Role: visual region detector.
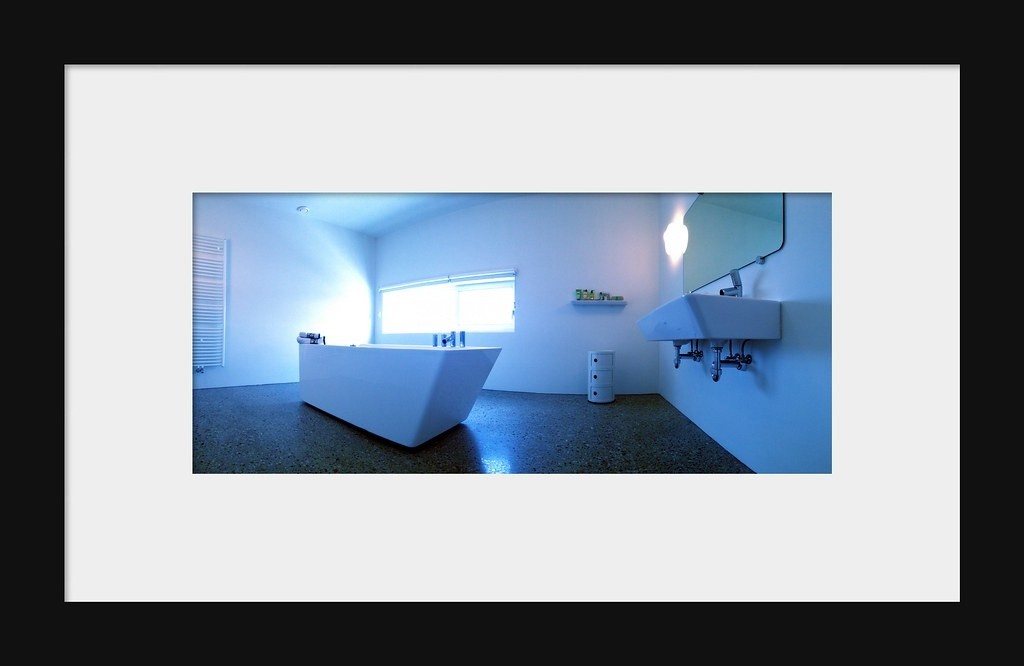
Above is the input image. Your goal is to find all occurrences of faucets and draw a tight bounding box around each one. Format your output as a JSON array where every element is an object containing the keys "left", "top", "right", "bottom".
[
  {"left": 719, "top": 268, "right": 743, "bottom": 298},
  {"left": 442, "top": 331, "right": 457, "bottom": 347}
]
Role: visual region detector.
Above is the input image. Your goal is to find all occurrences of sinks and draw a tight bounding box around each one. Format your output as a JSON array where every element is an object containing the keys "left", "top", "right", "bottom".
[{"left": 636, "top": 293, "right": 781, "bottom": 342}]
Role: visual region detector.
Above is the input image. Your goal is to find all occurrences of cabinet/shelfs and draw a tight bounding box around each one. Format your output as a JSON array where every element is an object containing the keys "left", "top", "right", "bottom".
[{"left": 587, "top": 349, "right": 616, "bottom": 403}]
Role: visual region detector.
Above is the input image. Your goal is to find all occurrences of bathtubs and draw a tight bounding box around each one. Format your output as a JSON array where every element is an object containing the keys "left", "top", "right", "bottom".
[{"left": 298, "top": 344, "right": 502, "bottom": 448}]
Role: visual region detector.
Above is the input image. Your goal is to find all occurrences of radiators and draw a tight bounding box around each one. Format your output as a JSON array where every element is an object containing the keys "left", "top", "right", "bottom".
[{"left": 192, "top": 231, "right": 227, "bottom": 374}]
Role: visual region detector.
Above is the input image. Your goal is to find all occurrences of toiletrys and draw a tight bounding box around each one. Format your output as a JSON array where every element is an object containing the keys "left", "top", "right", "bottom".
[{"left": 575, "top": 288, "right": 624, "bottom": 301}]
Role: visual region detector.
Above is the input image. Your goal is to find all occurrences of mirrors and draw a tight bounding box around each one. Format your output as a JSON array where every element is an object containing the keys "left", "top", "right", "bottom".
[{"left": 682, "top": 192, "right": 786, "bottom": 296}]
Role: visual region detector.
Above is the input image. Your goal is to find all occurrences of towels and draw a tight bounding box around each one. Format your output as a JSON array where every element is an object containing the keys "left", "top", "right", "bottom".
[{"left": 296, "top": 331, "right": 326, "bottom": 345}]
